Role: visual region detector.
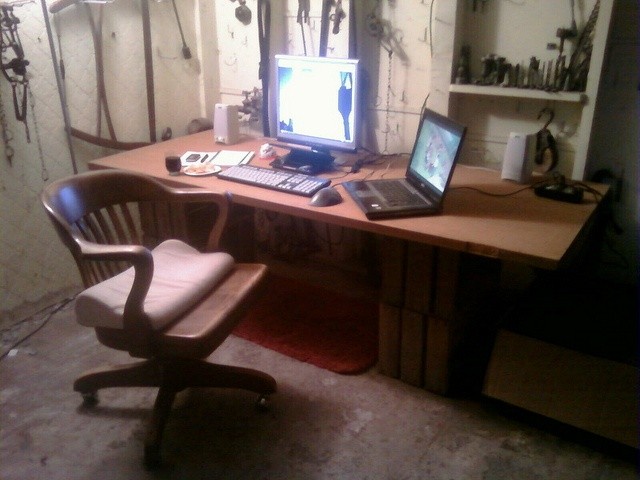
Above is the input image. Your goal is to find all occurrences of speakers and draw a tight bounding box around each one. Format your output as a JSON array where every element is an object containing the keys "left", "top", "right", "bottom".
[
  {"left": 214, "top": 103, "right": 240, "bottom": 146},
  {"left": 501, "top": 133, "right": 537, "bottom": 184}
]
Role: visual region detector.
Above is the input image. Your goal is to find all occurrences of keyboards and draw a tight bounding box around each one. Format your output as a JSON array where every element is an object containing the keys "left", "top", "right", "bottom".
[{"left": 217, "top": 164, "right": 331, "bottom": 198}]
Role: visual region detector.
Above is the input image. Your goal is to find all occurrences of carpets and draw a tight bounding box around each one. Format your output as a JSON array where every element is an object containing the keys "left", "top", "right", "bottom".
[{"left": 230, "top": 273, "right": 378, "bottom": 377}]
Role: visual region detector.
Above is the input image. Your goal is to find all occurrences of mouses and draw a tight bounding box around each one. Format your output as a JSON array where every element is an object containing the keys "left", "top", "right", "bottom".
[{"left": 310, "top": 186, "right": 342, "bottom": 207}]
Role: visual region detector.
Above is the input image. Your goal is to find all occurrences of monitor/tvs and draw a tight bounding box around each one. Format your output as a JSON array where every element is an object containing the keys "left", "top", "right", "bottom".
[{"left": 270, "top": 54, "right": 361, "bottom": 177}]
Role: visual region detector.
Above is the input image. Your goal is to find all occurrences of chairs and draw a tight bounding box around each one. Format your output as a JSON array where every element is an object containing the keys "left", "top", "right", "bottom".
[{"left": 38, "top": 169, "right": 278, "bottom": 472}]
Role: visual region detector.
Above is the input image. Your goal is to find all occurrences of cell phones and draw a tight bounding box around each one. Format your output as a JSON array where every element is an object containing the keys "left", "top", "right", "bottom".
[{"left": 186, "top": 153, "right": 200, "bottom": 162}]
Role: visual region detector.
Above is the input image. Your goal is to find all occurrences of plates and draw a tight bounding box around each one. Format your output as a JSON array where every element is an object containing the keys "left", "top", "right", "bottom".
[{"left": 182, "top": 164, "right": 221, "bottom": 176}]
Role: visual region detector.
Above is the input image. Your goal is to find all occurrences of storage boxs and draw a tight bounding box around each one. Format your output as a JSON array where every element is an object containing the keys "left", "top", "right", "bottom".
[{"left": 482, "top": 328, "right": 640, "bottom": 449}]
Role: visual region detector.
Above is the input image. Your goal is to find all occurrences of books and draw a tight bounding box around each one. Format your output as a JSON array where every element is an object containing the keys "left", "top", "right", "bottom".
[{"left": 212, "top": 150, "right": 254, "bottom": 166}]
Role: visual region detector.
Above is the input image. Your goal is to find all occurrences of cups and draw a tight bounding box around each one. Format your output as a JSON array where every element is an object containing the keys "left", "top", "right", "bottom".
[{"left": 164, "top": 152, "right": 182, "bottom": 178}]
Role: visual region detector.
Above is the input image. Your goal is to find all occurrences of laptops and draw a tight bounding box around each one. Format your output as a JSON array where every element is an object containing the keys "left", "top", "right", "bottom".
[{"left": 342, "top": 107, "right": 467, "bottom": 219}]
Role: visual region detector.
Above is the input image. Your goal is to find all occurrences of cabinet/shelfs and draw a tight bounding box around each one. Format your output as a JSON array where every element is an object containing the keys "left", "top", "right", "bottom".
[{"left": 427, "top": 0, "right": 616, "bottom": 182}]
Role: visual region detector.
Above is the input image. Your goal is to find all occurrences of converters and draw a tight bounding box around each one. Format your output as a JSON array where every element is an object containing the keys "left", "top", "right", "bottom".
[{"left": 534, "top": 183, "right": 582, "bottom": 203}]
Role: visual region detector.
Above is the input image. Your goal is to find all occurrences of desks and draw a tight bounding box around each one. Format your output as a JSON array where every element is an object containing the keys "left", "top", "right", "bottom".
[{"left": 86, "top": 126, "right": 612, "bottom": 396}]
